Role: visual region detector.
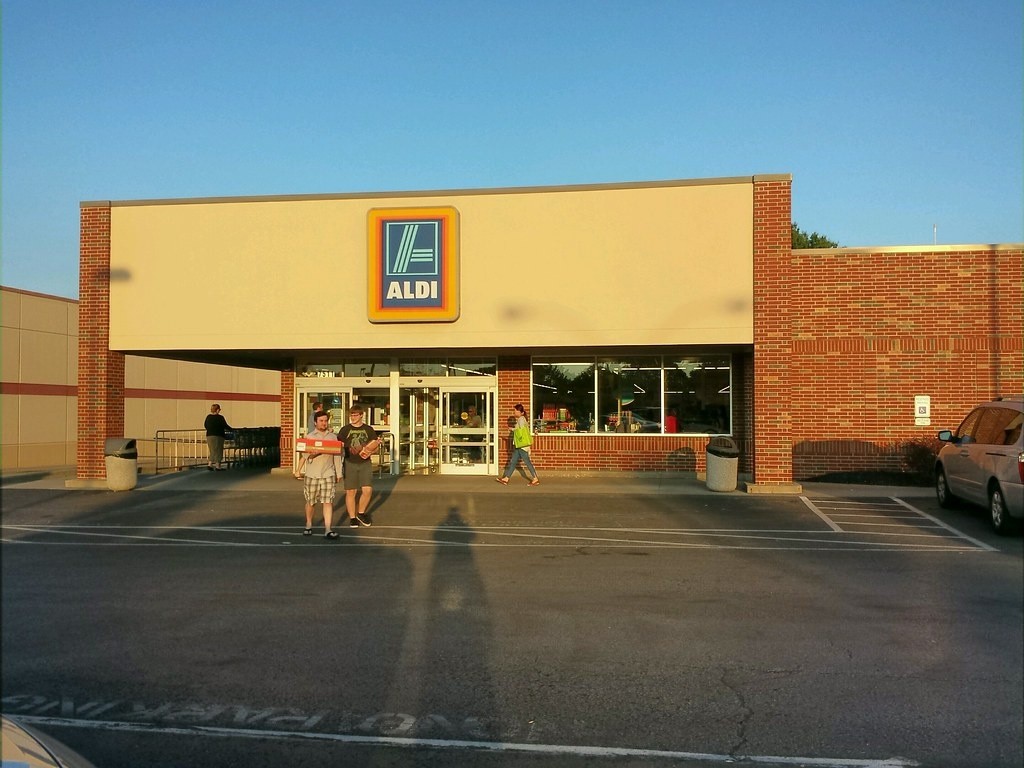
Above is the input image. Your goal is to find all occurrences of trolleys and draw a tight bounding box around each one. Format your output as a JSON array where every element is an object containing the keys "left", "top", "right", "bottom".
[{"left": 222, "top": 425, "right": 281, "bottom": 473}]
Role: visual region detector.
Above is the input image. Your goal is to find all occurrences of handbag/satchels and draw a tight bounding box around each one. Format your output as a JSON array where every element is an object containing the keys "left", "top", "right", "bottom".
[{"left": 514, "top": 426, "right": 534, "bottom": 449}]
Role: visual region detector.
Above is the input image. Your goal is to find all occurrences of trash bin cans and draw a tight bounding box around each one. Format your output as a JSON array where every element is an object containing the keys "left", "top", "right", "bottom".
[
  {"left": 706, "top": 436, "right": 739, "bottom": 492},
  {"left": 104, "top": 437, "right": 138, "bottom": 491}
]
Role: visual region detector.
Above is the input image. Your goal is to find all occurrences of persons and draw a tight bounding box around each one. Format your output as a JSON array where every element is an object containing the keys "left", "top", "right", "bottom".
[
  {"left": 338, "top": 405, "right": 376, "bottom": 529},
  {"left": 496, "top": 404, "right": 540, "bottom": 487},
  {"left": 294, "top": 402, "right": 324, "bottom": 481},
  {"left": 466, "top": 405, "right": 482, "bottom": 461},
  {"left": 301, "top": 411, "right": 344, "bottom": 538},
  {"left": 204, "top": 404, "right": 234, "bottom": 471}
]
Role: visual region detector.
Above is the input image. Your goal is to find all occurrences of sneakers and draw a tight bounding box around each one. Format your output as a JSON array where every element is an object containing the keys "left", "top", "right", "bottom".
[
  {"left": 350, "top": 518, "right": 359, "bottom": 528},
  {"left": 356, "top": 513, "right": 371, "bottom": 526}
]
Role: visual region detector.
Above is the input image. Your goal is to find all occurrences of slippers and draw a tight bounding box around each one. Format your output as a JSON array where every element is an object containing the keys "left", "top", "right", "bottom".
[
  {"left": 324, "top": 531, "right": 339, "bottom": 540},
  {"left": 293, "top": 476, "right": 304, "bottom": 481},
  {"left": 303, "top": 529, "right": 312, "bottom": 536}
]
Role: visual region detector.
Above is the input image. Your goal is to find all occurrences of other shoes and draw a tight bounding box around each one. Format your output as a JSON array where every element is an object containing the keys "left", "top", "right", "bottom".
[
  {"left": 216, "top": 468, "right": 221, "bottom": 471},
  {"left": 495, "top": 478, "right": 508, "bottom": 486},
  {"left": 527, "top": 480, "right": 540, "bottom": 486},
  {"left": 207, "top": 466, "right": 214, "bottom": 471}
]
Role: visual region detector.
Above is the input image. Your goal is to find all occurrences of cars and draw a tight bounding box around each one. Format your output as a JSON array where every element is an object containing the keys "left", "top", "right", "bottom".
[
  {"left": 933, "top": 392, "right": 1024, "bottom": 537},
  {"left": 599, "top": 409, "right": 661, "bottom": 433}
]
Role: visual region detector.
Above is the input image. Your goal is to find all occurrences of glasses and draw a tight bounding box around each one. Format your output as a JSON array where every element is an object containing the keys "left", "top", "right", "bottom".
[
  {"left": 350, "top": 414, "right": 360, "bottom": 417},
  {"left": 219, "top": 409, "right": 221, "bottom": 411}
]
